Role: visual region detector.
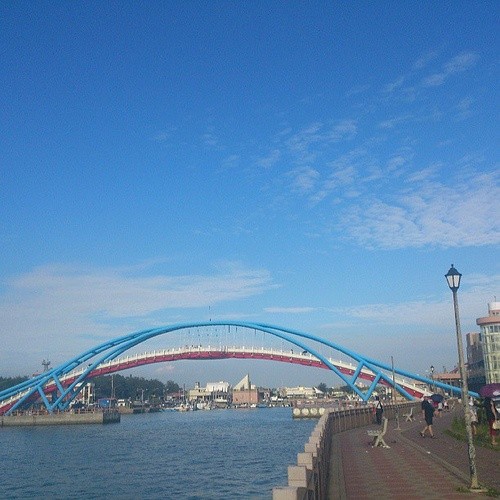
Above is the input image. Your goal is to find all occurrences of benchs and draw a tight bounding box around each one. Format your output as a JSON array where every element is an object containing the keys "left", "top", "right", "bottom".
[
  {"left": 403, "top": 406, "right": 416, "bottom": 424},
  {"left": 367, "top": 416, "right": 390, "bottom": 448}
]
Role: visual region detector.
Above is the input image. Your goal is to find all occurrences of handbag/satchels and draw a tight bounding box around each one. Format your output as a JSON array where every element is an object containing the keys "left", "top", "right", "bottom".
[
  {"left": 492, "top": 420, "right": 500, "bottom": 430},
  {"left": 373, "top": 407, "right": 376, "bottom": 414},
  {"left": 382, "top": 408, "right": 383, "bottom": 412}
]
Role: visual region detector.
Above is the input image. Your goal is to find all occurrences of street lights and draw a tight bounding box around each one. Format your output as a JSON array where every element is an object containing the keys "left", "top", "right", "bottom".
[
  {"left": 431, "top": 365, "right": 436, "bottom": 394},
  {"left": 444, "top": 263, "right": 483, "bottom": 490}
]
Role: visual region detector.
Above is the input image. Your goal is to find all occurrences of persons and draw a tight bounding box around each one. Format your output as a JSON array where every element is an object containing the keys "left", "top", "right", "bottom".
[
  {"left": 469, "top": 396, "right": 491, "bottom": 435},
  {"left": 420, "top": 393, "right": 435, "bottom": 439},
  {"left": 487, "top": 399, "right": 499, "bottom": 445},
  {"left": 432, "top": 400, "right": 449, "bottom": 418},
  {"left": 372, "top": 396, "right": 384, "bottom": 424}
]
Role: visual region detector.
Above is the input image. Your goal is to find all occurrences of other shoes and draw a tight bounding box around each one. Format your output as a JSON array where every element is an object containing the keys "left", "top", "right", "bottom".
[
  {"left": 431, "top": 435, "right": 438, "bottom": 439},
  {"left": 420, "top": 432, "right": 425, "bottom": 438}
]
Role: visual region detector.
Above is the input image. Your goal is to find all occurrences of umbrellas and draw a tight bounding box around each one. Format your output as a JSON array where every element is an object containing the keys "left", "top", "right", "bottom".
[{"left": 431, "top": 394, "right": 444, "bottom": 403}]
